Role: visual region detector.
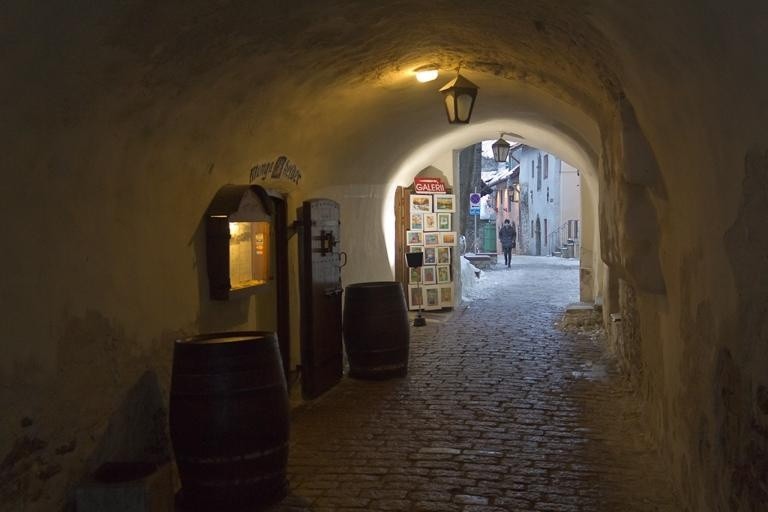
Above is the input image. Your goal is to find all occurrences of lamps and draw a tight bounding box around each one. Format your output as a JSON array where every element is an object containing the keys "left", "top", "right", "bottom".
[
  {"left": 439, "top": 65, "right": 482, "bottom": 126},
  {"left": 491, "top": 132, "right": 512, "bottom": 163}
]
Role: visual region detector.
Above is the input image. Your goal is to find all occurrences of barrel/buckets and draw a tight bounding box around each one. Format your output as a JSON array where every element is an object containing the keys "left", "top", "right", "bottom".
[
  {"left": 343, "top": 282, "right": 410, "bottom": 380},
  {"left": 169, "top": 331, "right": 290, "bottom": 512}
]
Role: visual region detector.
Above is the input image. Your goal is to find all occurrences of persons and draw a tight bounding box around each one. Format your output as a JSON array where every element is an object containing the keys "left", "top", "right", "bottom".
[{"left": 499, "top": 219, "right": 516, "bottom": 268}]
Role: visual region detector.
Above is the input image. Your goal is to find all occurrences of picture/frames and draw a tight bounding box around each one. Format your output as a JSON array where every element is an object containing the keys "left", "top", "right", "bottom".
[{"left": 405, "top": 193, "right": 459, "bottom": 313}]
